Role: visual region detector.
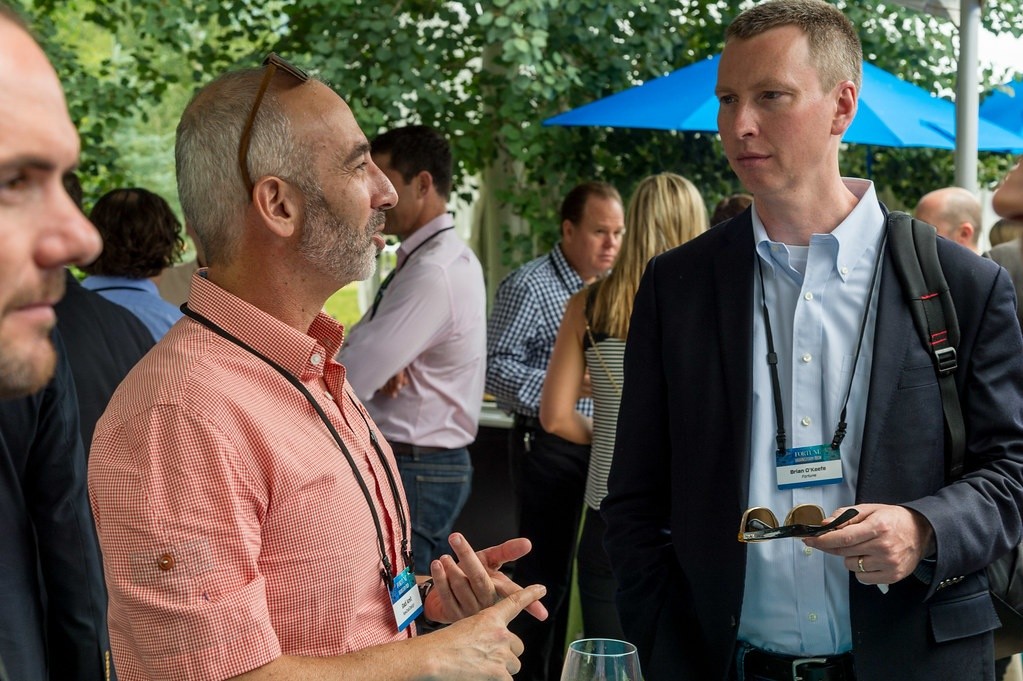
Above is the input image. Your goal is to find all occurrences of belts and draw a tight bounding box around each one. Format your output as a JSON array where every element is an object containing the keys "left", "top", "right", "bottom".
[{"left": 389, "top": 441, "right": 445, "bottom": 454}]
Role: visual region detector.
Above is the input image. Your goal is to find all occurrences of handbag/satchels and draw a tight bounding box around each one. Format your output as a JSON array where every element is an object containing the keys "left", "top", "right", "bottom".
[{"left": 886, "top": 211, "right": 1023, "bottom": 617}]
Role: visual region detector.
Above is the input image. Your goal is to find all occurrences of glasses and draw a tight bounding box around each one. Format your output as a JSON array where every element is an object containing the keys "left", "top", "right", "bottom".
[
  {"left": 737, "top": 504, "right": 860, "bottom": 543},
  {"left": 238, "top": 50, "right": 309, "bottom": 202}
]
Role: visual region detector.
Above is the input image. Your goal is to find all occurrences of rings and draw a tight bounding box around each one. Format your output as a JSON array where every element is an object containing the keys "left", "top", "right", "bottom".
[{"left": 858, "top": 556, "right": 867, "bottom": 572}]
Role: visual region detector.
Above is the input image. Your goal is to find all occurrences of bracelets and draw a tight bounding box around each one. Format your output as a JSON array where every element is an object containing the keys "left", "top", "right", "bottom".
[{"left": 420, "top": 579, "right": 434, "bottom": 602}]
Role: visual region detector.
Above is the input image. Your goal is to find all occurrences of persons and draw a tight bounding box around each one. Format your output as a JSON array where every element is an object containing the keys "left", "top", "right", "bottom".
[
  {"left": 334, "top": 127, "right": 486, "bottom": 574},
  {"left": 913, "top": 155, "right": 1023, "bottom": 329},
  {"left": 84, "top": 65, "right": 548, "bottom": 680},
  {"left": 598, "top": 0, "right": 1023, "bottom": 679},
  {"left": 0, "top": 12, "right": 187, "bottom": 680},
  {"left": 486, "top": 183, "right": 623, "bottom": 681},
  {"left": 538, "top": 172, "right": 712, "bottom": 643}
]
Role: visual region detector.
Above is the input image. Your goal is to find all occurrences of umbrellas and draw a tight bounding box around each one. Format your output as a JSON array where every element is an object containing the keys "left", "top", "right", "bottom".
[{"left": 544, "top": 54, "right": 1022, "bottom": 151}]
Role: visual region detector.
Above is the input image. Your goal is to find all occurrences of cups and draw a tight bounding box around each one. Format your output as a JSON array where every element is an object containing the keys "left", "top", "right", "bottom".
[{"left": 560, "top": 638, "right": 644, "bottom": 681}]
